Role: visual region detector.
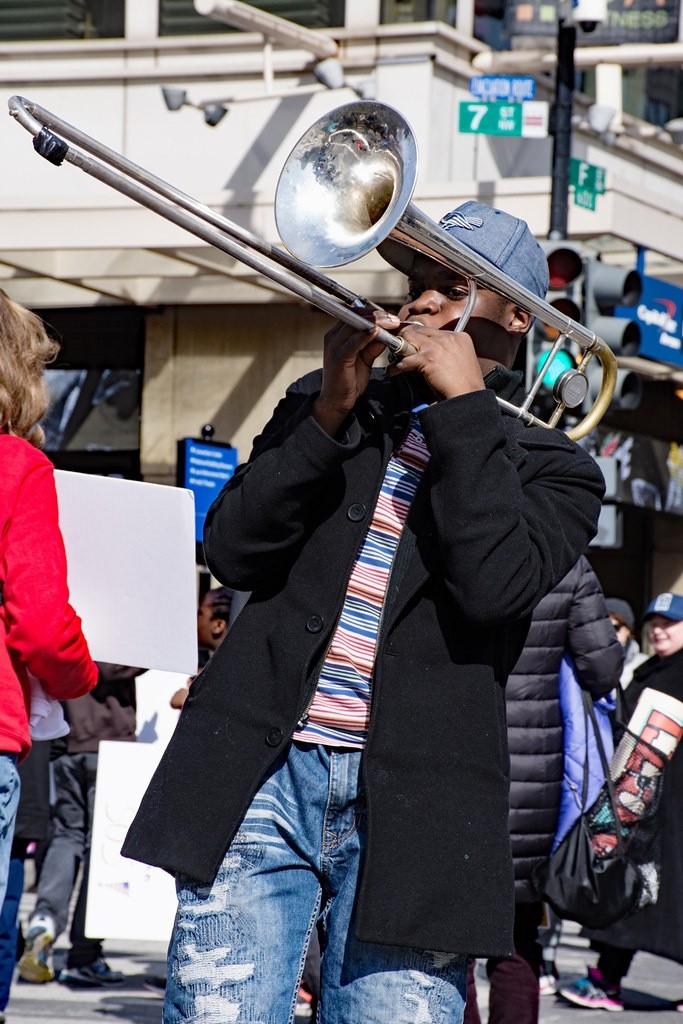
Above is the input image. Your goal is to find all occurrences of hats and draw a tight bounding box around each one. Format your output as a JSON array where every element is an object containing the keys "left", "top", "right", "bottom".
[
  {"left": 377, "top": 202, "right": 548, "bottom": 323},
  {"left": 640, "top": 591, "right": 682, "bottom": 622},
  {"left": 603, "top": 597, "right": 634, "bottom": 632}
]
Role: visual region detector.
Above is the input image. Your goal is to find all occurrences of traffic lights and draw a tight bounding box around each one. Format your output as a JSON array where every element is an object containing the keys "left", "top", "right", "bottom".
[
  {"left": 524, "top": 238, "right": 585, "bottom": 396},
  {"left": 587, "top": 449, "right": 624, "bottom": 551},
  {"left": 575, "top": 255, "right": 644, "bottom": 415}
]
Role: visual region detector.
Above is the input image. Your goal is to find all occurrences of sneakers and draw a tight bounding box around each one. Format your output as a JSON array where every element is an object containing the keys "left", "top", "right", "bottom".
[
  {"left": 58, "top": 956, "right": 128, "bottom": 986},
  {"left": 20, "top": 915, "right": 57, "bottom": 984},
  {"left": 558, "top": 965, "right": 624, "bottom": 1014}
]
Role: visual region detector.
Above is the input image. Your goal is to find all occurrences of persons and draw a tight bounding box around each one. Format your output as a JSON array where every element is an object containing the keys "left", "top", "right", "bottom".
[
  {"left": 461, "top": 555, "right": 683, "bottom": 1024},
  {"left": 120, "top": 200, "right": 607, "bottom": 1024},
  {"left": 0, "top": 281, "right": 321, "bottom": 1024}
]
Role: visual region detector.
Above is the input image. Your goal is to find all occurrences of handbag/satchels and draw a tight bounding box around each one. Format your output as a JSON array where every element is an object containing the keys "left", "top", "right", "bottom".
[{"left": 529, "top": 816, "right": 645, "bottom": 928}]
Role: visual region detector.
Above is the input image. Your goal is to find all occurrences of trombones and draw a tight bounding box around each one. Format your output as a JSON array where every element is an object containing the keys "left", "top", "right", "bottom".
[{"left": 7, "top": 95, "right": 619, "bottom": 443}]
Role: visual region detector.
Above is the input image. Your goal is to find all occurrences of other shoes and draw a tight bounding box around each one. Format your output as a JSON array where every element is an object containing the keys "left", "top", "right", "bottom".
[
  {"left": 538, "top": 974, "right": 558, "bottom": 994},
  {"left": 142, "top": 977, "right": 167, "bottom": 995}
]
